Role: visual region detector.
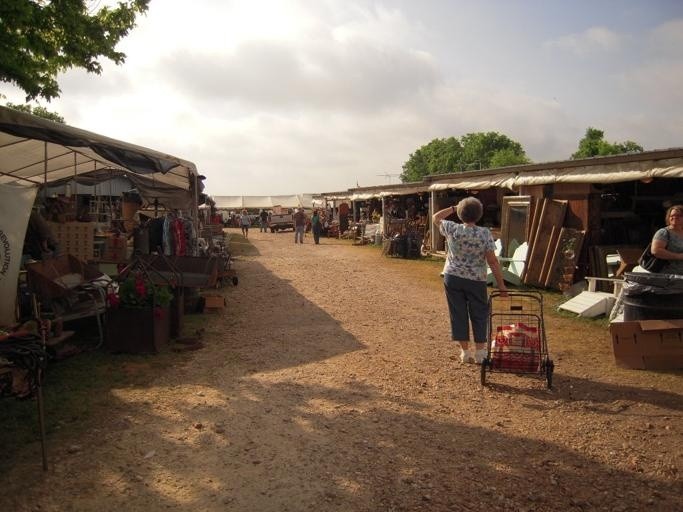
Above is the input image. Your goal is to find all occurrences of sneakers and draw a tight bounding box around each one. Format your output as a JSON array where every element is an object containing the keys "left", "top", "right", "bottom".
[
  {"left": 473, "top": 349, "right": 486, "bottom": 366},
  {"left": 459, "top": 349, "right": 470, "bottom": 363}
]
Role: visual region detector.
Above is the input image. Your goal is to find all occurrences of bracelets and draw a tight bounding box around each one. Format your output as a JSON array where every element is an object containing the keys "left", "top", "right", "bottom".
[{"left": 451, "top": 205, "right": 454, "bottom": 214}]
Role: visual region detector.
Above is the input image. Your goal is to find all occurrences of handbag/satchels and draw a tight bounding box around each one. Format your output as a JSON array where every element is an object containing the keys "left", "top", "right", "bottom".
[{"left": 638, "top": 228, "right": 671, "bottom": 273}]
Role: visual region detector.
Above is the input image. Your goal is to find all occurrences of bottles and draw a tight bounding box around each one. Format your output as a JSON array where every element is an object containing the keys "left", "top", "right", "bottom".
[{"left": 106, "top": 285, "right": 113, "bottom": 294}]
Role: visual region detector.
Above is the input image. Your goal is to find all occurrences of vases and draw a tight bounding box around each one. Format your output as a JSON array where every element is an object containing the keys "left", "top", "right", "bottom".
[{"left": 114, "top": 305, "right": 169, "bottom": 356}]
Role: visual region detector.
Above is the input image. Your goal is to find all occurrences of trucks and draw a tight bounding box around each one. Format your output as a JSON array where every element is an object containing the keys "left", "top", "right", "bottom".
[{"left": 224, "top": 207, "right": 295, "bottom": 233}]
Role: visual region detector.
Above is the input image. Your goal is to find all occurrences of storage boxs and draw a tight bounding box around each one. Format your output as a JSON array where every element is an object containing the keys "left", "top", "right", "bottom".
[
  {"left": 609, "top": 316, "right": 683, "bottom": 371},
  {"left": 199, "top": 293, "right": 224, "bottom": 314}
]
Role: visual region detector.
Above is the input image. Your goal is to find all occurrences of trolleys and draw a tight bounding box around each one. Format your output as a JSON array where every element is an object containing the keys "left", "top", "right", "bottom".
[
  {"left": 211, "top": 249, "right": 240, "bottom": 289},
  {"left": 478, "top": 288, "right": 555, "bottom": 390}
]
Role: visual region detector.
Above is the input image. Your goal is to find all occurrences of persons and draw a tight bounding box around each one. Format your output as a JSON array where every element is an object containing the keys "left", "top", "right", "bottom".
[
  {"left": 239, "top": 209, "right": 251, "bottom": 239},
  {"left": 310, "top": 210, "right": 323, "bottom": 244},
  {"left": 292, "top": 207, "right": 308, "bottom": 244},
  {"left": 25, "top": 210, "right": 67, "bottom": 260},
  {"left": 649, "top": 203, "right": 682, "bottom": 276},
  {"left": 258, "top": 209, "right": 269, "bottom": 233},
  {"left": 432, "top": 197, "right": 509, "bottom": 365}
]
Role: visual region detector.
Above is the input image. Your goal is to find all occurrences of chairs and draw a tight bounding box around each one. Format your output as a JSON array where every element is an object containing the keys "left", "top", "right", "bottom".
[{"left": 24, "top": 253, "right": 119, "bottom": 351}]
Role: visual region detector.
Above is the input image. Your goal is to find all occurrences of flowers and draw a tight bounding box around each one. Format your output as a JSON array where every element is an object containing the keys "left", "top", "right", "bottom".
[{"left": 106, "top": 267, "right": 177, "bottom": 322}]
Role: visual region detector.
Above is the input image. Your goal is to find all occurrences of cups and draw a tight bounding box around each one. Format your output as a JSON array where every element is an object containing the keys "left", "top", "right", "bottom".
[
  {"left": 52, "top": 317, "right": 62, "bottom": 337},
  {"left": 36, "top": 319, "right": 50, "bottom": 341}
]
